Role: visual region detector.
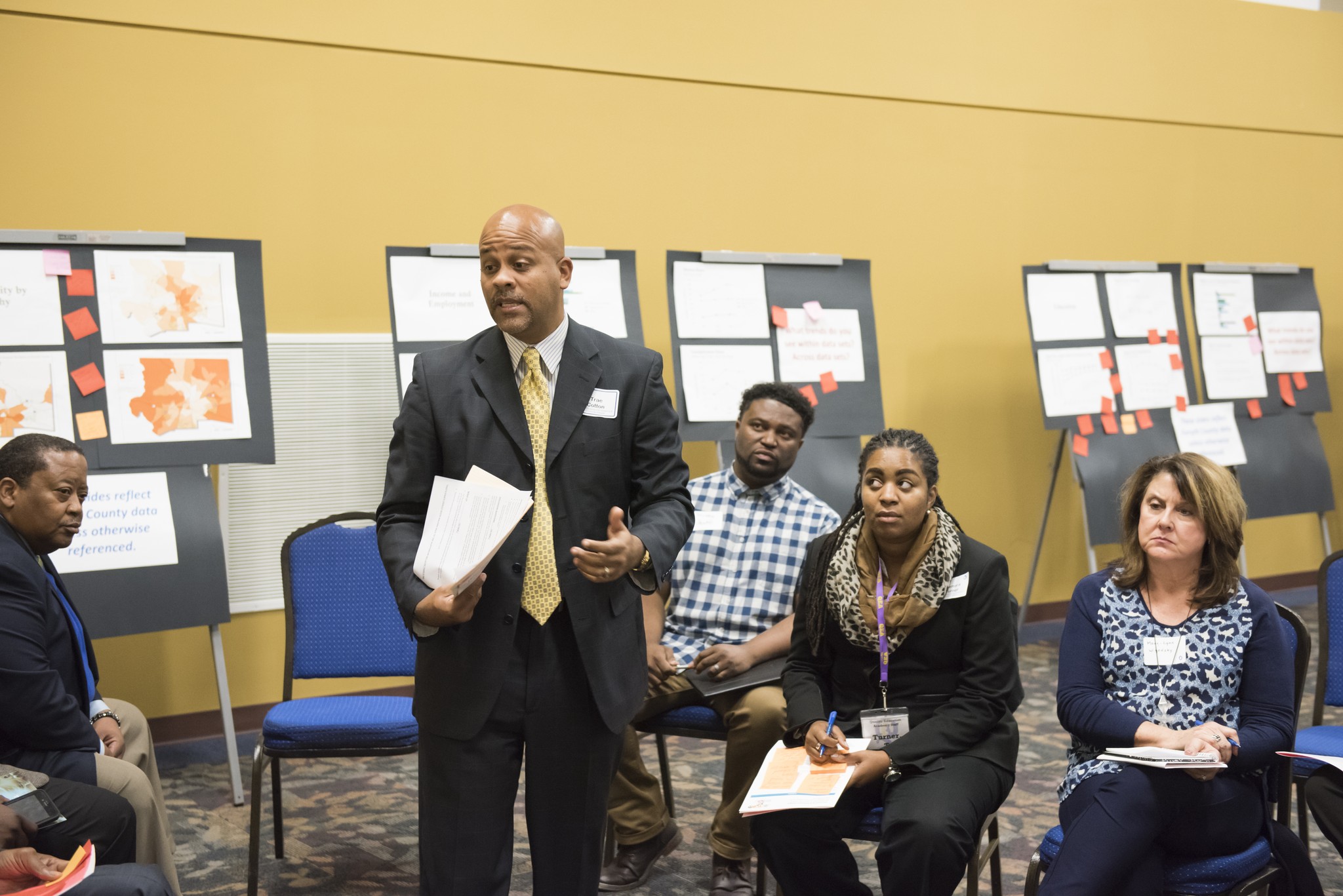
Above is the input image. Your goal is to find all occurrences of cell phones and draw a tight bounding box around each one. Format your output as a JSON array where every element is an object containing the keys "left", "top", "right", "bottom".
[{"left": 1, "top": 789, "right": 60, "bottom": 828}]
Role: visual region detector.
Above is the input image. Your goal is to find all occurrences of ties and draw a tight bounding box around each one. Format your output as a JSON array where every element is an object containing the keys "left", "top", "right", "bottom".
[
  {"left": 40, "top": 566, "right": 96, "bottom": 703},
  {"left": 515, "top": 346, "right": 563, "bottom": 625}
]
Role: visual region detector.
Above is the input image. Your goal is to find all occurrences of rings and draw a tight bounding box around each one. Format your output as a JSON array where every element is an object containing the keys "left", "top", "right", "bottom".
[
  {"left": 1212, "top": 733, "right": 1221, "bottom": 742},
  {"left": 603, "top": 565, "right": 610, "bottom": 580},
  {"left": 1200, "top": 775, "right": 1207, "bottom": 780},
  {"left": 713, "top": 663, "right": 723, "bottom": 674}
]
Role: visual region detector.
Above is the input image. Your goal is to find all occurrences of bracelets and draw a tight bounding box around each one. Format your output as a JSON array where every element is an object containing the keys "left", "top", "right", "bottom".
[{"left": 89, "top": 710, "right": 121, "bottom": 729}]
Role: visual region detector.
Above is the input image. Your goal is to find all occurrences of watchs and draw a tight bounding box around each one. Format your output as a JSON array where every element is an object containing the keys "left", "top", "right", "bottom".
[
  {"left": 632, "top": 550, "right": 652, "bottom": 573},
  {"left": 883, "top": 755, "right": 904, "bottom": 782}
]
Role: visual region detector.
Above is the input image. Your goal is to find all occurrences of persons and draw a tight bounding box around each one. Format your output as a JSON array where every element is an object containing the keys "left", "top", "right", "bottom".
[
  {"left": 746, "top": 430, "right": 1024, "bottom": 895},
  {"left": 0, "top": 433, "right": 183, "bottom": 893},
  {"left": 606, "top": 380, "right": 845, "bottom": 895},
  {"left": 0, "top": 761, "right": 138, "bottom": 863},
  {"left": 1032, "top": 448, "right": 1301, "bottom": 896},
  {"left": 371, "top": 200, "right": 698, "bottom": 894},
  {"left": 0, "top": 844, "right": 179, "bottom": 896}
]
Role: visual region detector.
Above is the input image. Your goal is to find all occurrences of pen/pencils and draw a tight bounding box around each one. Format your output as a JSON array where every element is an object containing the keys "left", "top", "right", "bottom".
[
  {"left": 819, "top": 711, "right": 837, "bottom": 758},
  {"left": 1194, "top": 719, "right": 1241, "bottom": 747},
  {"left": 670, "top": 664, "right": 696, "bottom": 668}
]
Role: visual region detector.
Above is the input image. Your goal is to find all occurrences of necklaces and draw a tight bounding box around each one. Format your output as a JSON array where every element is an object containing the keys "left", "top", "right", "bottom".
[{"left": 1146, "top": 582, "right": 1197, "bottom": 714}]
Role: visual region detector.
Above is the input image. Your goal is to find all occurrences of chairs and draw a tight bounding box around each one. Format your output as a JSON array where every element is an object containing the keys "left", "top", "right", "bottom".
[
  {"left": 754, "top": 596, "right": 1024, "bottom": 895},
  {"left": 245, "top": 514, "right": 421, "bottom": 896},
  {"left": 1001, "top": 602, "right": 1312, "bottom": 895},
  {"left": 645, "top": 699, "right": 734, "bottom": 814},
  {"left": 1279, "top": 547, "right": 1343, "bottom": 850}
]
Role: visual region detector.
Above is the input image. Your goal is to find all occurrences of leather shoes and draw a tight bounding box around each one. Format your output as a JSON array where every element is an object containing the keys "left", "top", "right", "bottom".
[
  {"left": 709, "top": 850, "right": 754, "bottom": 896},
  {"left": 597, "top": 817, "right": 683, "bottom": 890}
]
